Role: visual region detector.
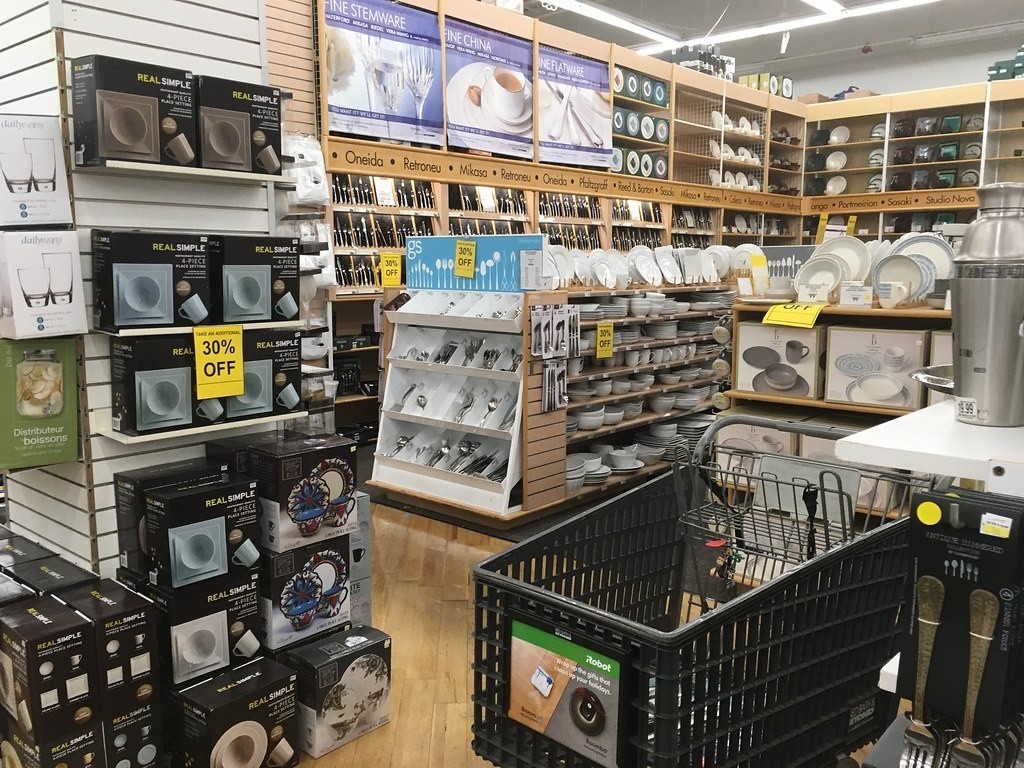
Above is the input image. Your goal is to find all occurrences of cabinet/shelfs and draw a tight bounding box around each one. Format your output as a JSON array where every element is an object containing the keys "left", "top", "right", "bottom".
[
  {"left": 294, "top": 0, "right": 1024, "bottom": 453},
  {"left": 709, "top": 279, "right": 955, "bottom": 532},
  {"left": 362, "top": 276, "right": 736, "bottom": 536},
  {"left": 63, "top": 54, "right": 311, "bottom": 447}
]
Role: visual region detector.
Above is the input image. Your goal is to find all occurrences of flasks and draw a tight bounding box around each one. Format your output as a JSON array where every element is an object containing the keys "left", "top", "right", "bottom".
[{"left": 948, "top": 177, "right": 1024, "bottom": 430}]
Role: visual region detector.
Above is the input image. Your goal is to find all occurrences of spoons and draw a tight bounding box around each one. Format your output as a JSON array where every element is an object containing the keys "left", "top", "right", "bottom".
[{"left": 332, "top": 173, "right": 530, "bottom": 487}]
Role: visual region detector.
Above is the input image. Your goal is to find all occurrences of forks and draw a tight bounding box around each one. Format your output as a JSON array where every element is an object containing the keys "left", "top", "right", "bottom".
[{"left": 894, "top": 572, "right": 1024, "bottom": 768}]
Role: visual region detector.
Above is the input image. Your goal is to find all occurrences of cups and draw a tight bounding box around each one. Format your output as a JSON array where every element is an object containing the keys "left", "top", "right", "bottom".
[
  {"left": 482, "top": 63, "right": 527, "bottom": 120},
  {"left": 6, "top": 72, "right": 392, "bottom": 768}
]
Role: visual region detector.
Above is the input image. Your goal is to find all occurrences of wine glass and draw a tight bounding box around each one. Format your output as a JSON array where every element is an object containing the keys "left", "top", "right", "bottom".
[{"left": 357, "top": 32, "right": 436, "bottom": 141}]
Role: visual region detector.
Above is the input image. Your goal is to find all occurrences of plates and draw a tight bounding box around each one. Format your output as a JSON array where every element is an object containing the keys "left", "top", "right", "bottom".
[
  {"left": 537, "top": 66, "right": 984, "bottom": 496},
  {"left": 447, "top": 60, "right": 534, "bottom": 159}
]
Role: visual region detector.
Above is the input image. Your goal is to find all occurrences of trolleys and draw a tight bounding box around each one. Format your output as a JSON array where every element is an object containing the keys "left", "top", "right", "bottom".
[{"left": 467, "top": 412, "right": 933, "bottom": 768}]
